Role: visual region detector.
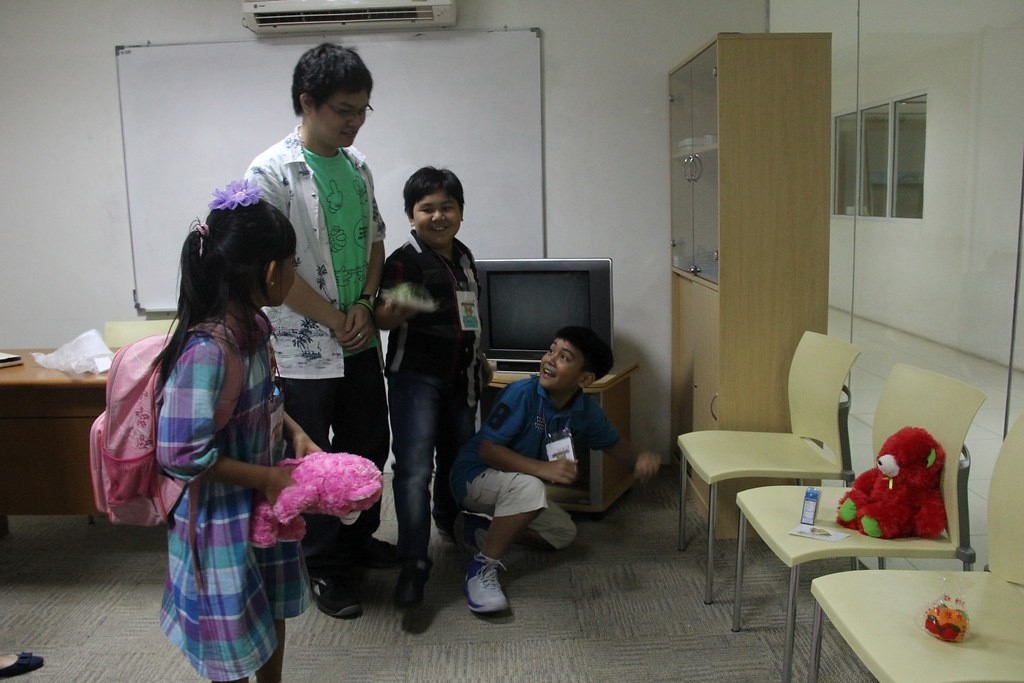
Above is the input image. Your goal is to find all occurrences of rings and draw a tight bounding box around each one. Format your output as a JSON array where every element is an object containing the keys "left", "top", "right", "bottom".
[{"left": 358, "top": 333, "right": 364, "bottom": 340}]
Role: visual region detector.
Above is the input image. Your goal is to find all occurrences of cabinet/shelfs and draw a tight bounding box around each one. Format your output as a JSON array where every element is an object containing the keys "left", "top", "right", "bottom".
[
  {"left": 669, "top": 32, "right": 831, "bottom": 540},
  {"left": 0, "top": 347, "right": 120, "bottom": 540}
]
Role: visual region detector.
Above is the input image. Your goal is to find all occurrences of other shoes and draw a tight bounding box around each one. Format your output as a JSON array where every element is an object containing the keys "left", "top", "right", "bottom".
[
  {"left": 0, "top": 651, "right": 43, "bottom": 677},
  {"left": 435, "top": 518, "right": 455, "bottom": 538},
  {"left": 395, "top": 560, "right": 428, "bottom": 609}
]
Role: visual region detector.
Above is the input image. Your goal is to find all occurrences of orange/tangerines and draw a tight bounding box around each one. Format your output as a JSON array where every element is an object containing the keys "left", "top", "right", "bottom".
[{"left": 926, "top": 606, "right": 967, "bottom": 641}]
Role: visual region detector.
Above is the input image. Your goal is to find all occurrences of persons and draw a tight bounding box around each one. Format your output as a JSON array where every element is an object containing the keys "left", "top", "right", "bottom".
[
  {"left": 454, "top": 327, "right": 659, "bottom": 613},
  {"left": 378, "top": 168, "right": 490, "bottom": 609},
  {"left": 160, "top": 180, "right": 323, "bottom": 683},
  {"left": 245, "top": 44, "right": 408, "bottom": 617},
  {"left": 0, "top": 651, "right": 44, "bottom": 678}
]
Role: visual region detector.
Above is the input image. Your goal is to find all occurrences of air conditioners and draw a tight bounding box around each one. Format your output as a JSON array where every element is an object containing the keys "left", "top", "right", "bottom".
[{"left": 242, "top": 0, "right": 456, "bottom": 34}]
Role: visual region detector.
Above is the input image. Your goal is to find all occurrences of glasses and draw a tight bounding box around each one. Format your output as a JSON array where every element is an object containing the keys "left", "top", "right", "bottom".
[{"left": 325, "top": 101, "right": 374, "bottom": 117}]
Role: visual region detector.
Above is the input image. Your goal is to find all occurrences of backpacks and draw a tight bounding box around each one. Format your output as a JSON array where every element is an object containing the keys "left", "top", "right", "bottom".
[{"left": 89, "top": 315, "right": 243, "bottom": 528}]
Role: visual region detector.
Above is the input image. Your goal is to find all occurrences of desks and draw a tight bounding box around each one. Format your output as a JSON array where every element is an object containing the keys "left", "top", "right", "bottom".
[{"left": 479, "top": 358, "right": 641, "bottom": 511}]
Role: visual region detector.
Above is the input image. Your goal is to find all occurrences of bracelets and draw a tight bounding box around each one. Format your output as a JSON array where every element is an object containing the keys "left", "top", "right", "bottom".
[{"left": 356, "top": 301, "right": 374, "bottom": 318}]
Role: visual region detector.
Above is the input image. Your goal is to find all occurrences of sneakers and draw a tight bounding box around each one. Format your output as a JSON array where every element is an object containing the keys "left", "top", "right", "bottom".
[
  {"left": 351, "top": 539, "right": 401, "bottom": 568},
  {"left": 465, "top": 556, "right": 508, "bottom": 612},
  {"left": 310, "top": 573, "right": 361, "bottom": 617},
  {"left": 454, "top": 511, "right": 480, "bottom": 558}
]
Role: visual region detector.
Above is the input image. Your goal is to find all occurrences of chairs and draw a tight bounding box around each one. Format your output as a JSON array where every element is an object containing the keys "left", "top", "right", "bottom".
[
  {"left": 88, "top": 320, "right": 180, "bottom": 523},
  {"left": 678, "top": 331, "right": 861, "bottom": 605},
  {"left": 731, "top": 364, "right": 988, "bottom": 683},
  {"left": 806, "top": 411, "right": 1024, "bottom": 683}
]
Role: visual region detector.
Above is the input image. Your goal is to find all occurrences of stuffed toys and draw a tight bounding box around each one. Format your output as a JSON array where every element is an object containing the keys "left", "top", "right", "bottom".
[
  {"left": 839, "top": 426, "right": 948, "bottom": 538},
  {"left": 249, "top": 452, "right": 385, "bottom": 548}
]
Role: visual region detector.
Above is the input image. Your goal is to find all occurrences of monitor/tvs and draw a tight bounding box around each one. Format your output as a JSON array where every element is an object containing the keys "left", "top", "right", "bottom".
[{"left": 474, "top": 257, "right": 614, "bottom": 374}]
